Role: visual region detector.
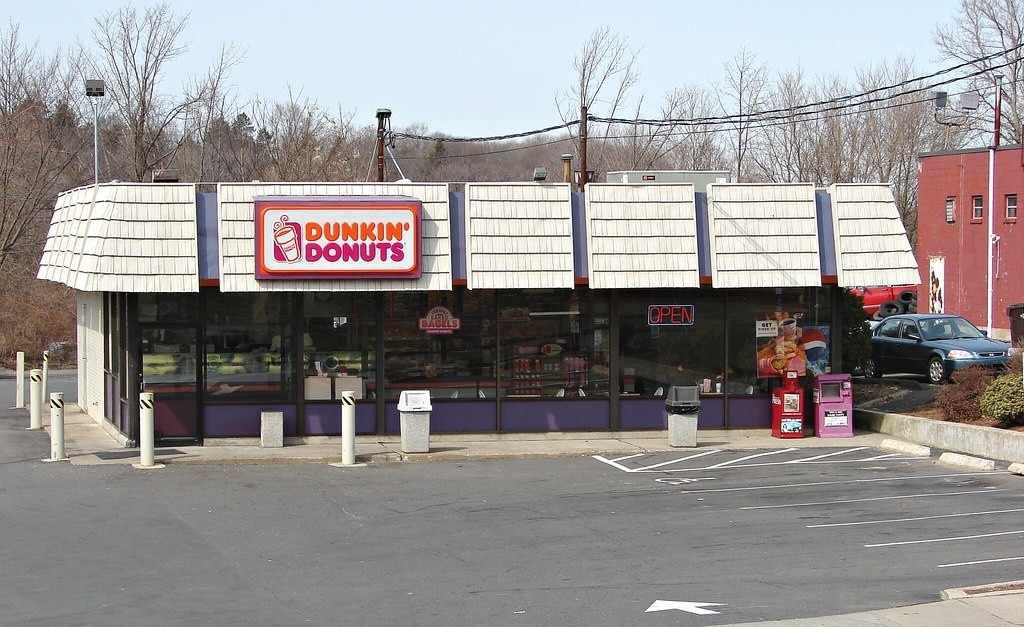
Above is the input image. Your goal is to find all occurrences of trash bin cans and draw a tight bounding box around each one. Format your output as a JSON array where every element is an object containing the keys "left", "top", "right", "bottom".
[
  {"left": 397, "top": 388, "right": 432, "bottom": 456},
  {"left": 1006, "top": 304, "right": 1024, "bottom": 348},
  {"left": 666, "top": 385, "right": 703, "bottom": 452}
]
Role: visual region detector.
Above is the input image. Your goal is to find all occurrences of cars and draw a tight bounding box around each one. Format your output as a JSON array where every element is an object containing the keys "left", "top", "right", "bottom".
[
  {"left": 863, "top": 314, "right": 1016, "bottom": 384},
  {"left": 848, "top": 284, "right": 917, "bottom": 315}
]
